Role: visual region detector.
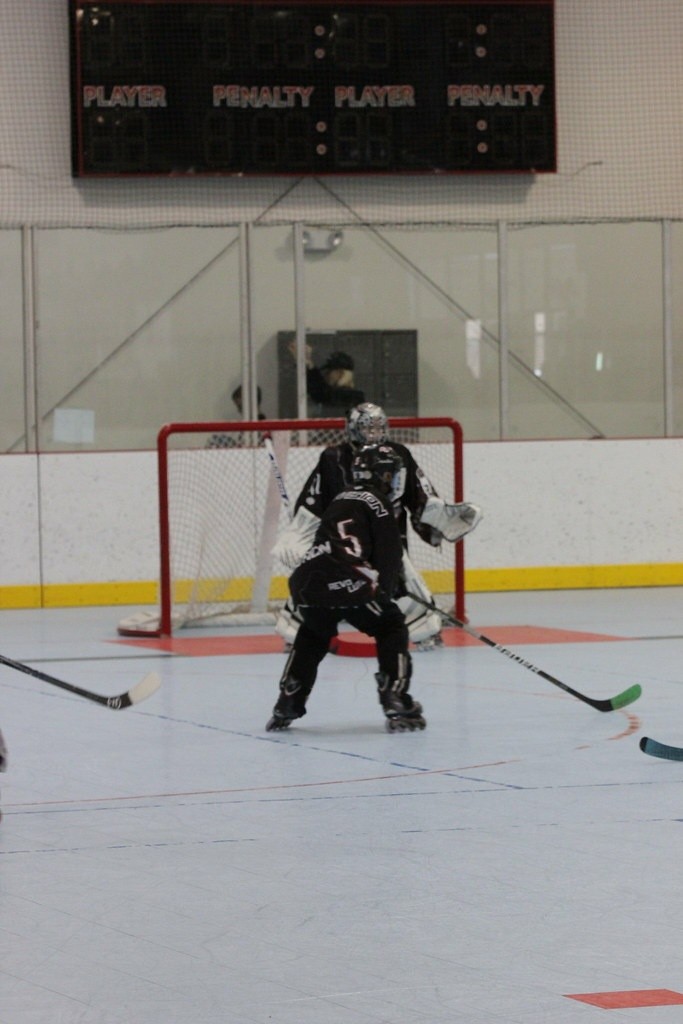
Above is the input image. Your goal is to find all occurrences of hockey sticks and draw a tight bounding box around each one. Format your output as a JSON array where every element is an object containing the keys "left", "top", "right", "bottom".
[
  {"left": 404, "top": 590, "right": 643, "bottom": 714},
  {"left": 1, "top": 655, "right": 163, "bottom": 712},
  {"left": 638, "top": 735, "right": 683, "bottom": 762},
  {"left": 260, "top": 430, "right": 297, "bottom": 526}
]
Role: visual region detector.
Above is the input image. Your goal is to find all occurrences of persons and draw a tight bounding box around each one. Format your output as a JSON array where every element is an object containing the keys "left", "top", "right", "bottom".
[
  {"left": 207, "top": 384, "right": 272, "bottom": 450},
  {"left": 264, "top": 444, "right": 426, "bottom": 733},
  {"left": 273, "top": 401, "right": 481, "bottom": 650},
  {"left": 289, "top": 339, "right": 364, "bottom": 443}
]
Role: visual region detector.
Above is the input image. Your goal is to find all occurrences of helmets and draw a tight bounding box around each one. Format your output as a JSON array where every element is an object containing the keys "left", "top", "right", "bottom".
[
  {"left": 350, "top": 444, "right": 406, "bottom": 503},
  {"left": 343, "top": 402, "right": 390, "bottom": 451}
]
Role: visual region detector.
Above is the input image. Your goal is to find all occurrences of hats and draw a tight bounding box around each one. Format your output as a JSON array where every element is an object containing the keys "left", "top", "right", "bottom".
[{"left": 317, "top": 350, "right": 354, "bottom": 373}]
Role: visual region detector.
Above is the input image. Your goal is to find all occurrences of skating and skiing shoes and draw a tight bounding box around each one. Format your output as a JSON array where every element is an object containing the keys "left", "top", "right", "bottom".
[
  {"left": 374, "top": 672, "right": 426, "bottom": 732},
  {"left": 266, "top": 679, "right": 312, "bottom": 732}
]
